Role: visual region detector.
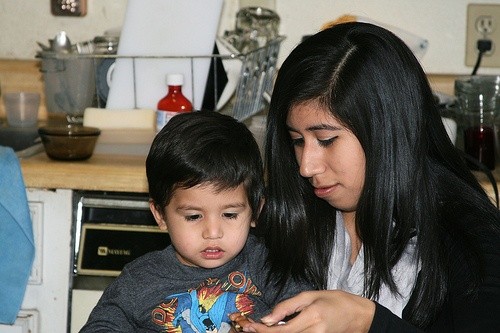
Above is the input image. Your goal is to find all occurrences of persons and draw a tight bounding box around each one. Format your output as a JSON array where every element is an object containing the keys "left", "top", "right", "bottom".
[
  {"left": 78, "top": 111, "right": 318, "bottom": 333},
  {"left": 242, "top": 21, "right": 499, "bottom": 333}
]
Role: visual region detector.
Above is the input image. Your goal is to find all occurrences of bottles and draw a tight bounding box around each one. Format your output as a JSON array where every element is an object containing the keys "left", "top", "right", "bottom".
[{"left": 153, "top": 74, "right": 193, "bottom": 136}]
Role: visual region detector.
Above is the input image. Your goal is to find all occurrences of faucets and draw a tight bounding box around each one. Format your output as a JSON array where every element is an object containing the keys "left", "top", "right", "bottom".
[{"left": 433, "top": 92, "right": 500, "bottom": 168}]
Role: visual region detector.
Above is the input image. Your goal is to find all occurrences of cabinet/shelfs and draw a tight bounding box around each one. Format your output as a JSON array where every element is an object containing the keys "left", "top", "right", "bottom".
[{"left": 0, "top": 164, "right": 172, "bottom": 333}]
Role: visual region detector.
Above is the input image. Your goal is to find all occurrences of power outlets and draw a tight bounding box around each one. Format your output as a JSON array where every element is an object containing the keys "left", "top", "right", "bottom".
[{"left": 464, "top": 3, "right": 500, "bottom": 67}]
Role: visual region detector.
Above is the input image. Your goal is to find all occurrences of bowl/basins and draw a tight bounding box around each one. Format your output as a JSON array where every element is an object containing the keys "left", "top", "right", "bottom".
[
  {"left": 39, "top": 126, "right": 102, "bottom": 162},
  {"left": 2, "top": 93, "right": 41, "bottom": 127}
]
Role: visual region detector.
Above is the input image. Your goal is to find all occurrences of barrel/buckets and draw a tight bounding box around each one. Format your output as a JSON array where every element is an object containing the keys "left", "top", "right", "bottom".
[{"left": 36, "top": 52, "right": 103, "bottom": 117}]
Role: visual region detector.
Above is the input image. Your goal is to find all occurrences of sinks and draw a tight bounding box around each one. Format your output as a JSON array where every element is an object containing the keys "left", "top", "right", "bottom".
[{"left": 1, "top": 129, "right": 43, "bottom": 152}]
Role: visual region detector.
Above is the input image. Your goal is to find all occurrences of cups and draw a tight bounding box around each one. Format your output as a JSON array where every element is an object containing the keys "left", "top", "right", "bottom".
[{"left": 450, "top": 76, "right": 500, "bottom": 172}]
